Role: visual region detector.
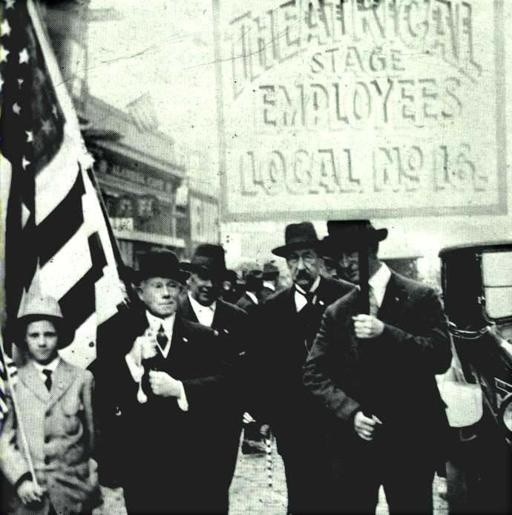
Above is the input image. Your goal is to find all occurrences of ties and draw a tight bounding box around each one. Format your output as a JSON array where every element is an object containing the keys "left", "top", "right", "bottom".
[
  {"left": 157, "top": 325, "right": 167, "bottom": 351},
  {"left": 294, "top": 288, "right": 316, "bottom": 320},
  {"left": 43, "top": 369, "right": 51, "bottom": 391},
  {"left": 367, "top": 287, "right": 379, "bottom": 316}
]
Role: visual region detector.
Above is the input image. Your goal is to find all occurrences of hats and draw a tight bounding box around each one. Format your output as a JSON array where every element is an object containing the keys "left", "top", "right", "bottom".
[
  {"left": 7, "top": 295, "right": 75, "bottom": 350},
  {"left": 130, "top": 251, "right": 191, "bottom": 282},
  {"left": 246, "top": 270, "right": 264, "bottom": 285},
  {"left": 180, "top": 244, "right": 237, "bottom": 279},
  {"left": 272, "top": 222, "right": 322, "bottom": 257},
  {"left": 312, "top": 220, "right": 388, "bottom": 256},
  {"left": 261, "top": 263, "right": 281, "bottom": 273}
]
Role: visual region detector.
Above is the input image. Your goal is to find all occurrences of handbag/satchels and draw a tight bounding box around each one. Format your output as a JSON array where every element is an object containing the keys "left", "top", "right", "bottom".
[{"left": 436, "top": 366, "right": 484, "bottom": 428}]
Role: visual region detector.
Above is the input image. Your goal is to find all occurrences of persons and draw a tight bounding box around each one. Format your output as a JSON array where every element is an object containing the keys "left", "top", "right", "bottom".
[{"left": 1, "top": 218, "right": 511, "bottom": 515}]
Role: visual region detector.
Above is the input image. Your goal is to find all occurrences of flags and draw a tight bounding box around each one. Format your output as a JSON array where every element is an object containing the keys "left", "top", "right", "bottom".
[{"left": 0, "top": 0, "right": 126, "bottom": 381}]
